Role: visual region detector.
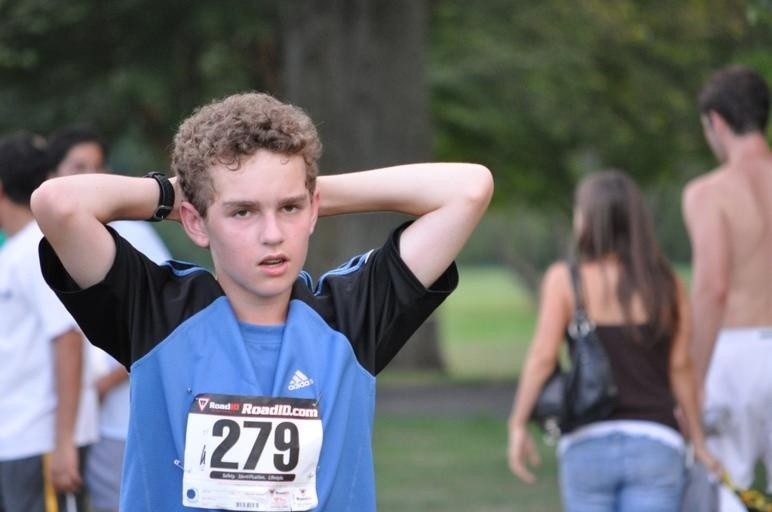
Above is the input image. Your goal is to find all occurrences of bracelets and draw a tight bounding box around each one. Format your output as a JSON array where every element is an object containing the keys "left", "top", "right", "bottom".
[{"left": 140, "top": 170, "right": 175, "bottom": 223}]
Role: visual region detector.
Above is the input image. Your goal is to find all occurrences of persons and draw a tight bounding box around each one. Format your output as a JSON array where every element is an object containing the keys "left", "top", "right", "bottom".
[
  {"left": 672, "top": 65, "right": 770, "bottom": 510},
  {"left": 1, "top": 125, "right": 102, "bottom": 511},
  {"left": 29, "top": 89, "right": 495, "bottom": 512},
  {"left": 40, "top": 125, "right": 174, "bottom": 512},
  {"left": 503, "top": 167, "right": 727, "bottom": 511}
]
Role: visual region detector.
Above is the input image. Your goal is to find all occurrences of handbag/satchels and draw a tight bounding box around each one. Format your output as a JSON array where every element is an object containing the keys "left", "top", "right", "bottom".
[{"left": 526, "top": 257, "right": 611, "bottom": 430}]
